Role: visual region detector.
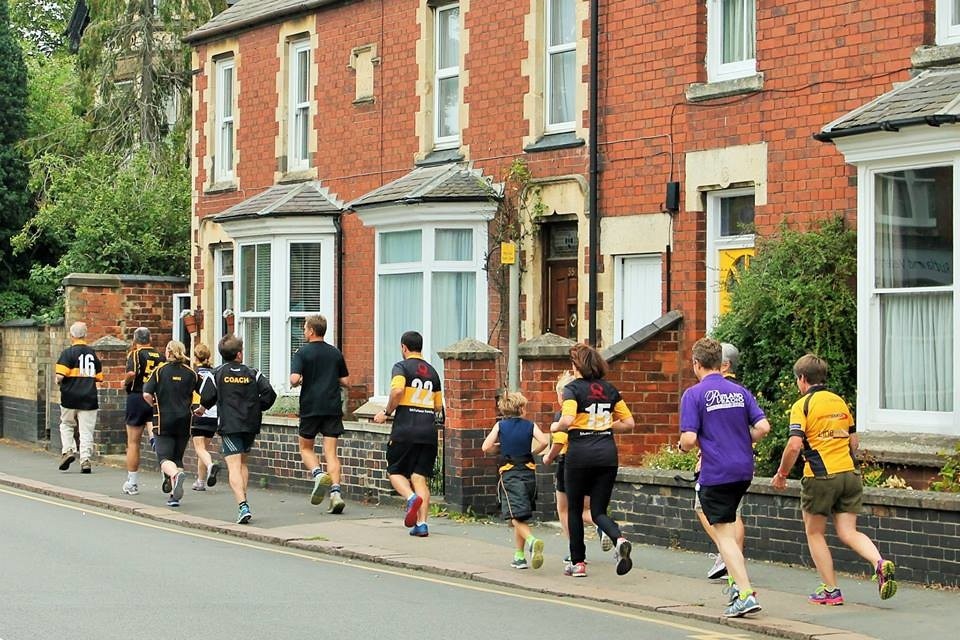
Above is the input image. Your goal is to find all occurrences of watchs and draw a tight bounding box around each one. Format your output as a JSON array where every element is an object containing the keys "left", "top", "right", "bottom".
[
  {"left": 677, "top": 442, "right": 688, "bottom": 454},
  {"left": 382, "top": 407, "right": 392, "bottom": 418}
]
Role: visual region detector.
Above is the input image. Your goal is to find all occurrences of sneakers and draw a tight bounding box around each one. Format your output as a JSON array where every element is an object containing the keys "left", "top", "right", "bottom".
[
  {"left": 595, "top": 526, "right": 612, "bottom": 552},
  {"left": 871, "top": 559, "right": 898, "bottom": 600},
  {"left": 528, "top": 538, "right": 545, "bottom": 570},
  {"left": 123, "top": 481, "right": 139, "bottom": 495},
  {"left": 162, "top": 472, "right": 172, "bottom": 494},
  {"left": 409, "top": 523, "right": 429, "bottom": 537},
  {"left": 808, "top": 582, "right": 844, "bottom": 606},
  {"left": 172, "top": 472, "right": 185, "bottom": 500},
  {"left": 59, "top": 451, "right": 75, "bottom": 470},
  {"left": 562, "top": 555, "right": 587, "bottom": 564},
  {"left": 326, "top": 494, "right": 345, "bottom": 514},
  {"left": 81, "top": 461, "right": 91, "bottom": 473},
  {"left": 613, "top": 538, "right": 633, "bottom": 576},
  {"left": 206, "top": 464, "right": 219, "bottom": 487},
  {"left": 192, "top": 480, "right": 205, "bottom": 491},
  {"left": 310, "top": 474, "right": 332, "bottom": 505},
  {"left": 510, "top": 556, "right": 527, "bottom": 569},
  {"left": 236, "top": 504, "right": 252, "bottom": 524},
  {"left": 564, "top": 561, "right": 587, "bottom": 577},
  {"left": 722, "top": 589, "right": 762, "bottom": 618},
  {"left": 723, "top": 576, "right": 739, "bottom": 607},
  {"left": 166, "top": 494, "right": 180, "bottom": 506},
  {"left": 707, "top": 553, "right": 728, "bottom": 580},
  {"left": 404, "top": 494, "right": 423, "bottom": 527}
]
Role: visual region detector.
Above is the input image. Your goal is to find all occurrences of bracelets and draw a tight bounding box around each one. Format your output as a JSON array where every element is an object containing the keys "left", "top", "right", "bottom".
[{"left": 776, "top": 466, "right": 789, "bottom": 479}]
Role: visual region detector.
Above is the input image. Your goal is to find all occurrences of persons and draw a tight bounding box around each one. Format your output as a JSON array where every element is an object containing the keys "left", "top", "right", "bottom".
[
  {"left": 190, "top": 342, "right": 222, "bottom": 491},
  {"left": 479, "top": 390, "right": 550, "bottom": 571},
  {"left": 121, "top": 323, "right": 174, "bottom": 495},
  {"left": 54, "top": 321, "right": 102, "bottom": 474},
  {"left": 142, "top": 339, "right": 206, "bottom": 505},
  {"left": 540, "top": 371, "right": 616, "bottom": 564},
  {"left": 372, "top": 329, "right": 446, "bottom": 537},
  {"left": 290, "top": 313, "right": 352, "bottom": 514},
  {"left": 673, "top": 336, "right": 775, "bottom": 618},
  {"left": 548, "top": 342, "right": 635, "bottom": 578},
  {"left": 767, "top": 353, "right": 900, "bottom": 608},
  {"left": 691, "top": 341, "right": 748, "bottom": 586},
  {"left": 197, "top": 334, "right": 278, "bottom": 525}
]
